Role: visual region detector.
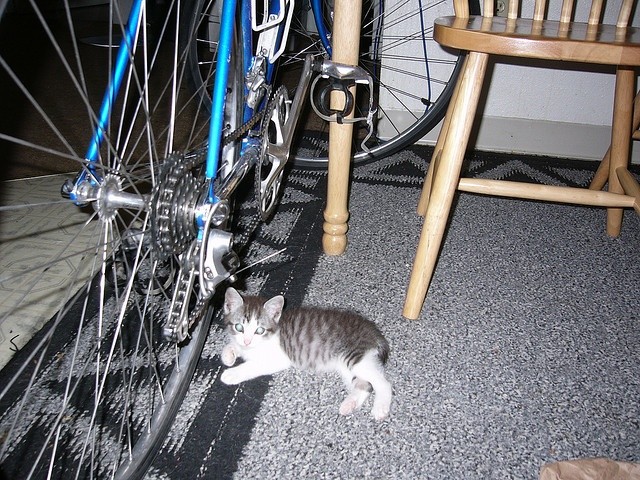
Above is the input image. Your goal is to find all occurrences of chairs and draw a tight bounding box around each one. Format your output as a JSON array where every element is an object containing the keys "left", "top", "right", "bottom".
[{"left": 400, "top": 0, "right": 639, "bottom": 320}]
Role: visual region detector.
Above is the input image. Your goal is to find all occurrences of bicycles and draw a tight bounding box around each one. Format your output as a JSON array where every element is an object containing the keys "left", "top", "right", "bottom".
[{"left": 0, "top": 0, "right": 487, "bottom": 480}]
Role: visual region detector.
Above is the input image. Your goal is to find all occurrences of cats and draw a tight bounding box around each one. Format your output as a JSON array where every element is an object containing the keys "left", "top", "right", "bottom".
[{"left": 220, "top": 286, "right": 393, "bottom": 424}]
